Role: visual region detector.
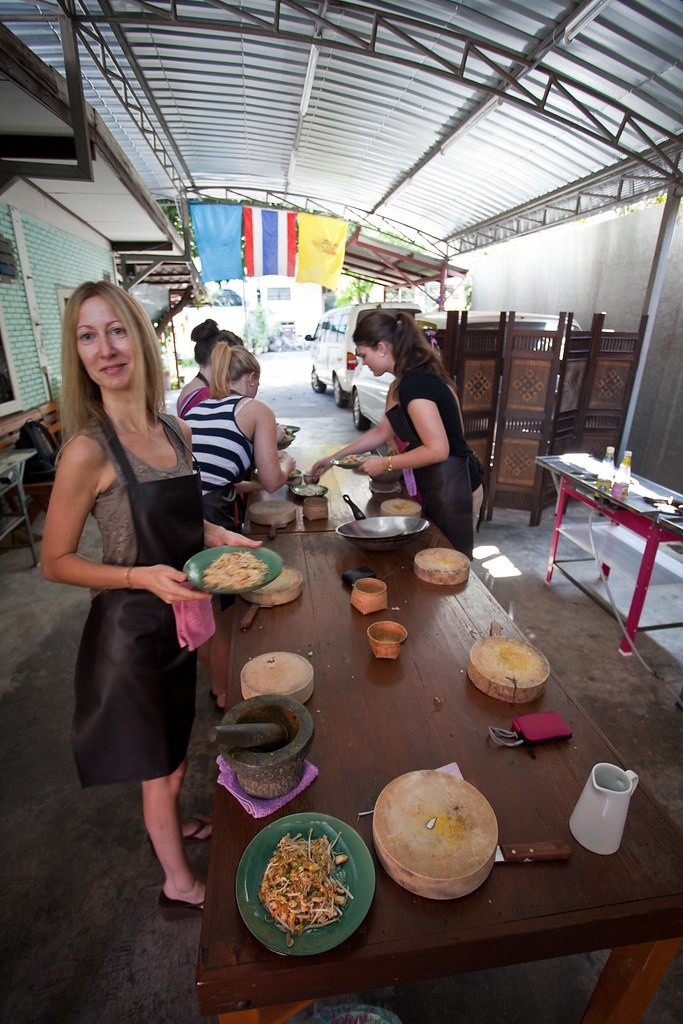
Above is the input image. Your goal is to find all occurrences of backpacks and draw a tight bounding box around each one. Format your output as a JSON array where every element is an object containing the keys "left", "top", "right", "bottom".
[{"left": 15, "top": 418, "right": 60, "bottom": 474}]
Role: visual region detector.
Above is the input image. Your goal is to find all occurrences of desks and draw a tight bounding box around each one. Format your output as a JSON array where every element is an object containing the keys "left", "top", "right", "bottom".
[
  {"left": 534, "top": 453, "right": 682, "bottom": 661},
  {"left": 242, "top": 443, "right": 416, "bottom": 530},
  {"left": 192, "top": 520, "right": 683, "bottom": 1024},
  {"left": 0, "top": 448, "right": 41, "bottom": 567}
]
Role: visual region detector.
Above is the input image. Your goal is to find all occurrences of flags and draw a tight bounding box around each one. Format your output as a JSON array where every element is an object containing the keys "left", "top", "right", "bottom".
[
  {"left": 298, "top": 212, "right": 348, "bottom": 290},
  {"left": 245, "top": 206, "right": 296, "bottom": 277},
  {"left": 189, "top": 201, "right": 244, "bottom": 281}
]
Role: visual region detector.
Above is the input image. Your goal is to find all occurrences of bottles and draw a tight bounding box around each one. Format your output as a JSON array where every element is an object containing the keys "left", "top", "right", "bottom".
[
  {"left": 593, "top": 446, "right": 615, "bottom": 498},
  {"left": 609, "top": 450, "right": 632, "bottom": 501}
]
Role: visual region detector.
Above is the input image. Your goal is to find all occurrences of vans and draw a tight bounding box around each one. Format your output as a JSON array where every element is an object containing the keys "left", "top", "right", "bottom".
[
  {"left": 305, "top": 301, "right": 423, "bottom": 408},
  {"left": 351, "top": 313, "right": 583, "bottom": 432}
]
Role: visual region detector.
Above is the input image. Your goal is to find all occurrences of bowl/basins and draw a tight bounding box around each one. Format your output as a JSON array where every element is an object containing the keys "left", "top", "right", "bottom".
[
  {"left": 286, "top": 475, "right": 302, "bottom": 487},
  {"left": 303, "top": 473, "right": 319, "bottom": 484}
]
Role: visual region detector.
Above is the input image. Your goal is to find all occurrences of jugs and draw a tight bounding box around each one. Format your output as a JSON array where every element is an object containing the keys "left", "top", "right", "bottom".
[{"left": 569, "top": 763, "right": 639, "bottom": 855}]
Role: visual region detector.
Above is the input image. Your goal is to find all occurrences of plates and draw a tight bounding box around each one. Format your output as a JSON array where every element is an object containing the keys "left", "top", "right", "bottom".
[
  {"left": 290, "top": 483, "right": 328, "bottom": 498},
  {"left": 330, "top": 454, "right": 378, "bottom": 469},
  {"left": 235, "top": 813, "right": 376, "bottom": 956},
  {"left": 253, "top": 468, "right": 301, "bottom": 479},
  {"left": 183, "top": 546, "right": 285, "bottom": 595},
  {"left": 276, "top": 426, "right": 300, "bottom": 448}
]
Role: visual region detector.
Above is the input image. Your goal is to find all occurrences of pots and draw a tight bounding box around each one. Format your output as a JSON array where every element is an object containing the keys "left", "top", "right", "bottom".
[{"left": 335, "top": 495, "right": 431, "bottom": 551}]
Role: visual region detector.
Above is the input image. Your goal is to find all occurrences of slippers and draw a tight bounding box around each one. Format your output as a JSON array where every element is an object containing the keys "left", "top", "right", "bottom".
[
  {"left": 149, "top": 814, "right": 212, "bottom": 842},
  {"left": 158, "top": 886, "right": 205, "bottom": 912}
]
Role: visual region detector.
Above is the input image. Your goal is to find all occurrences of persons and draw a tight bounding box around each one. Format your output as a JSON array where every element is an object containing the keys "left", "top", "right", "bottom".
[
  {"left": 40, "top": 283, "right": 263, "bottom": 911},
  {"left": 177, "top": 319, "right": 295, "bottom": 716},
  {"left": 310, "top": 311, "right": 484, "bottom": 562}
]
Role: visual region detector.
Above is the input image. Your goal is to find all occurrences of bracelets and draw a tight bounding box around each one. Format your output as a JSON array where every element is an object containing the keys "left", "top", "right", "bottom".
[
  {"left": 386, "top": 456, "right": 392, "bottom": 474},
  {"left": 125, "top": 566, "right": 133, "bottom": 589}
]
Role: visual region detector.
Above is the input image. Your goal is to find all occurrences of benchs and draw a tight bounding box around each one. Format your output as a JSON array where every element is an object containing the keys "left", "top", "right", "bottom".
[{"left": 0, "top": 400, "right": 67, "bottom": 515}]
